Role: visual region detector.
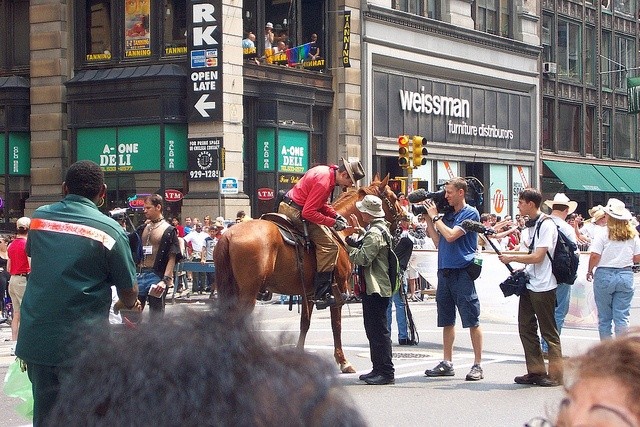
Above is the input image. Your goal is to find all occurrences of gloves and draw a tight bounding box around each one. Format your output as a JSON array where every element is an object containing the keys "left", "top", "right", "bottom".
[
  {"left": 333, "top": 219, "right": 347, "bottom": 231},
  {"left": 335, "top": 213, "right": 348, "bottom": 224}
]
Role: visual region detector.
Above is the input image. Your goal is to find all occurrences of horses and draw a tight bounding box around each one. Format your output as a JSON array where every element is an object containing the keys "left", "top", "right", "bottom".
[{"left": 213, "top": 171, "right": 411, "bottom": 373}]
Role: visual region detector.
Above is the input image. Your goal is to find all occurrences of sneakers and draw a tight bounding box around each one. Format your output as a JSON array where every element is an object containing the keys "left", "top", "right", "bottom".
[
  {"left": 364, "top": 372, "right": 395, "bottom": 384},
  {"left": 11, "top": 346, "right": 16, "bottom": 355},
  {"left": 543, "top": 349, "right": 570, "bottom": 360},
  {"left": 398, "top": 336, "right": 412, "bottom": 344},
  {"left": 359, "top": 369, "right": 377, "bottom": 379},
  {"left": 465, "top": 363, "right": 483, "bottom": 380},
  {"left": 514, "top": 372, "right": 547, "bottom": 383},
  {"left": 425, "top": 361, "right": 455, "bottom": 376},
  {"left": 0, "top": 314, "right": 7, "bottom": 323},
  {"left": 537, "top": 374, "right": 564, "bottom": 386}
]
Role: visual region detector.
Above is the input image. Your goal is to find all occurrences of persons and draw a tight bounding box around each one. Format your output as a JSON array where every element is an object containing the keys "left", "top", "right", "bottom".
[
  {"left": 480, "top": 212, "right": 519, "bottom": 251},
  {"left": 350, "top": 194, "right": 396, "bottom": 385},
  {"left": 397, "top": 192, "right": 409, "bottom": 213},
  {"left": 541, "top": 192, "right": 578, "bottom": 359},
  {"left": 242, "top": 31, "right": 256, "bottom": 49},
  {"left": 0, "top": 235, "right": 11, "bottom": 319},
  {"left": 184, "top": 216, "right": 193, "bottom": 237},
  {"left": 265, "top": 22, "right": 275, "bottom": 48},
  {"left": 516, "top": 219, "right": 526, "bottom": 232},
  {"left": 237, "top": 210, "right": 246, "bottom": 218},
  {"left": 387, "top": 273, "right": 410, "bottom": 345},
  {"left": 273, "top": 41, "right": 286, "bottom": 54},
  {"left": 121, "top": 221, "right": 127, "bottom": 231},
  {"left": 193, "top": 217, "right": 200, "bottom": 229},
  {"left": 37, "top": 303, "right": 368, "bottom": 426},
  {"left": 215, "top": 217, "right": 224, "bottom": 226},
  {"left": 236, "top": 218, "right": 241, "bottom": 224},
  {"left": 183, "top": 222, "right": 210, "bottom": 293},
  {"left": 575, "top": 210, "right": 606, "bottom": 246},
  {"left": 200, "top": 225, "right": 220, "bottom": 292},
  {"left": 490, "top": 214, "right": 511, "bottom": 231},
  {"left": 171, "top": 216, "right": 184, "bottom": 237},
  {"left": 520, "top": 330, "right": 639, "bottom": 426},
  {"left": 277, "top": 156, "right": 365, "bottom": 312},
  {"left": 523, "top": 214, "right": 530, "bottom": 222},
  {"left": 422, "top": 176, "right": 484, "bottom": 381},
  {"left": 515, "top": 214, "right": 520, "bottom": 224},
  {"left": 308, "top": 32, "right": 320, "bottom": 60},
  {"left": 587, "top": 197, "right": 640, "bottom": 340},
  {"left": 565, "top": 214, "right": 576, "bottom": 227},
  {"left": 14, "top": 159, "right": 140, "bottom": 427},
  {"left": 413, "top": 214, "right": 427, "bottom": 249},
  {"left": 202, "top": 215, "right": 211, "bottom": 232},
  {"left": 400, "top": 218, "right": 421, "bottom": 303},
  {"left": 7, "top": 217, "right": 32, "bottom": 342},
  {"left": 176, "top": 228, "right": 187, "bottom": 297},
  {"left": 497, "top": 189, "right": 566, "bottom": 387},
  {"left": 119, "top": 193, "right": 181, "bottom": 324}
]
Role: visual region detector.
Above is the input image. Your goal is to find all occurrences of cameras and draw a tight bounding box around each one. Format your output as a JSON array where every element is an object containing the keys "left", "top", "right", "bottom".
[
  {"left": 107, "top": 206, "right": 128, "bottom": 224},
  {"left": 406, "top": 177, "right": 484, "bottom": 216},
  {"left": 343, "top": 233, "right": 364, "bottom": 253}
]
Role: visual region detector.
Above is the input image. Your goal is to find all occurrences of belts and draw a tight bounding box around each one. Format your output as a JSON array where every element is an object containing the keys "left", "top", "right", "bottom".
[
  {"left": 13, "top": 272, "right": 27, "bottom": 276},
  {"left": 283, "top": 197, "right": 302, "bottom": 210}
]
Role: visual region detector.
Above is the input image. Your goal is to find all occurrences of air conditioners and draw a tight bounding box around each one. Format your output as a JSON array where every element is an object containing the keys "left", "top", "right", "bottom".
[{"left": 542, "top": 62, "right": 557, "bottom": 73}]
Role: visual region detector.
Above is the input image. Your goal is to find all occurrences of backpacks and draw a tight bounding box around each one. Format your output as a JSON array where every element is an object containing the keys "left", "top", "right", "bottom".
[{"left": 537, "top": 214, "right": 579, "bottom": 284}]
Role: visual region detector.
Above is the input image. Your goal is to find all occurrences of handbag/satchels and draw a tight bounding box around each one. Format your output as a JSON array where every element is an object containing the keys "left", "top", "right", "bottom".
[{"left": 388, "top": 248, "right": 402, "bottom": 295}]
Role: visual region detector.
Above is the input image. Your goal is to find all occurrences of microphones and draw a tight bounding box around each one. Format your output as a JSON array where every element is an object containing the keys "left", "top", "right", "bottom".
[{"left": 461, "top": 220, "right": 495, "bottom": 234}]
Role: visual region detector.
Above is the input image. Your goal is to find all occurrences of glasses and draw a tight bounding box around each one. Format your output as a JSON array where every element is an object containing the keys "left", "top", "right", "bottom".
[
  {"left": 236, "top": 221, "right": 240, "bottom": 223},
  {"left": 204, "top": 218, "right": 209, "bottom": 220},
  {"left": 0, "top": 240, "right": 3, "bottom": 242}
]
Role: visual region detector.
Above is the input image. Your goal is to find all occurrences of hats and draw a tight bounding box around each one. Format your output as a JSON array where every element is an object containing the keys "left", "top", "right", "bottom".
[
  {"left": 213, "top": 221, "right": 224, "bottom": 228},
  {"left": 341, "top": 156, "right": 365, "bottom": 186},
  {"left": 602, "top": 197, "right": 633, "bottom": 219},
  {"left": 590, "top": 209, "right": 605, "bottom": 222},
  {"left": 355, "top": 194, "right": 386, "bottom": 218},
  {"left": 544, "top": 191, "right": 578, "bottom": 214},
  {"left": 17, "top": 216, "right": 31, "bottom": 229}
]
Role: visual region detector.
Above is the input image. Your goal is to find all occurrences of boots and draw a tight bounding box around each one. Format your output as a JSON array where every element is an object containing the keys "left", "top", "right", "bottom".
[{"left": 314, "top": 270, "right": 347, "bottom": 309}]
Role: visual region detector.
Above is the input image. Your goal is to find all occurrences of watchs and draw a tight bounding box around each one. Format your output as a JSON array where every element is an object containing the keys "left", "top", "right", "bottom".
[{"left": 433, "top": 215, "right": 441, "bottom": 223}]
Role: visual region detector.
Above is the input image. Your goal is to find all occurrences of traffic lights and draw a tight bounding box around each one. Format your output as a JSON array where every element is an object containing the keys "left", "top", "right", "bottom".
[
  {"left": 398, "top": 135, "right": 410, "bottom": 167},
  {"left": 413, "top": 136, "right": 428, "bottom": 166},
  {"left": 413, "top": 180, "right": 429, "bottom": 191},
  {"left": 387, "top": 179, "right": 405, "bottom": 196}
]
